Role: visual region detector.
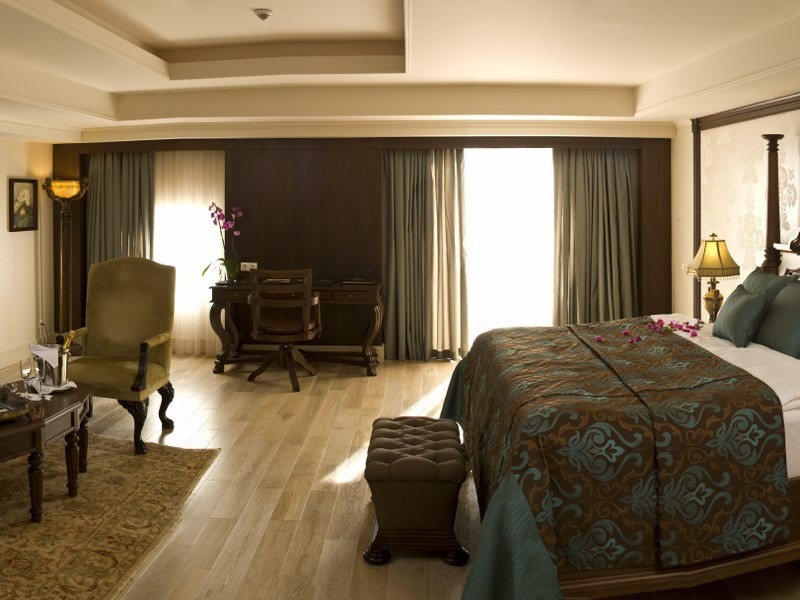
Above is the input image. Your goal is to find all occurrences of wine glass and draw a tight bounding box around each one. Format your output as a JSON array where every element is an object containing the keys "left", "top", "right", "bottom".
[{"left": 19, "top": 358, "right": 36, "bottom": 398}]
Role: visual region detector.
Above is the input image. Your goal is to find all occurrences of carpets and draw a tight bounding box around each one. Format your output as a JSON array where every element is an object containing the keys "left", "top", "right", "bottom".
[{"left": 0, "top": 431, "right": 222, "bottom": 600}]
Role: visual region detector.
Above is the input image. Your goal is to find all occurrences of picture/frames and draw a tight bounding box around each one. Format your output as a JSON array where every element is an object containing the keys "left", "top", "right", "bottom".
[{"left": 9, "top": 178, "right": 39, "bottom": 232}]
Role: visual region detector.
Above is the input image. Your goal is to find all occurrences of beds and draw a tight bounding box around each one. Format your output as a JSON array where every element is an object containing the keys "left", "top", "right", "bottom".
[{"left": 439, "top": 134, "right": 800, "bottom": 600}]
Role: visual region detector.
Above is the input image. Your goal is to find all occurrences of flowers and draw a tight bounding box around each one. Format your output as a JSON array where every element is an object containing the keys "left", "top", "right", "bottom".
[
  {"left": 595, "top": 319, "right": 705, "bottom": 343},
  {"left": 201, "top": 202, "right": 244, "bottom": 278}
]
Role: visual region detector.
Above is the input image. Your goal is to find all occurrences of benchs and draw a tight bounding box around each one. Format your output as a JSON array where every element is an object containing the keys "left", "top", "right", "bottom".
[{"left": 361, "top": 415, "right": 472, "bottom": 565}]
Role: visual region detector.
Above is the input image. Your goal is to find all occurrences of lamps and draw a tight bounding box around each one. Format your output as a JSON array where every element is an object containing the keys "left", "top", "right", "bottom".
[
  {"left": 684, "top": 233, "right": 740, "bottom": 323},
  {"left": 41, "top": 176, "right": 90, "bottom": 343}
]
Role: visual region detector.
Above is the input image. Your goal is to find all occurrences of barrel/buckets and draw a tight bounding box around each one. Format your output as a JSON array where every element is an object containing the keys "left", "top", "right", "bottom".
[{"left": 33, "top": 347, "right": 70, "bottom": 388}]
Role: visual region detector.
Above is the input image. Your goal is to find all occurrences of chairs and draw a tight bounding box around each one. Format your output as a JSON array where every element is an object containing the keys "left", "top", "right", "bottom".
[
  {"left": 247, "top": 268, "right": 322, "bottom": 392},
  {"left": 57, "top": 255, "right": 176, "bottom": 453}
]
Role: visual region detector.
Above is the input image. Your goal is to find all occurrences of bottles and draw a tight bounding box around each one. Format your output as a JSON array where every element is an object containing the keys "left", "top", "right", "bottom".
[{"left": 60, "top": 329, "right": 76, "bottom": 350}]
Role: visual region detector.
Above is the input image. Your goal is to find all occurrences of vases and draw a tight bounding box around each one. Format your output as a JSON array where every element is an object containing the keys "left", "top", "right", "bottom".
[{"left": 224, "top": 261, "right": 240, "bottom": 283}]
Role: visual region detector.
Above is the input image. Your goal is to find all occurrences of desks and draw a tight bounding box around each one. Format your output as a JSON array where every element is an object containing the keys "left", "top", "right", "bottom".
[
  {"left": 210, "top": 280, "right": 385, "bottom": 376},
  {"left": 0, "top": 379, "right": 94, "bottom": 523}
]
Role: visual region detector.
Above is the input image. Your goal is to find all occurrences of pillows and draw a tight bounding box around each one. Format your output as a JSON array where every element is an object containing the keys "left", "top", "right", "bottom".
[
  {"left": 743, "top": 266, "right": 800, "bottom": 306},
  {"left": 713, "top": 284, "right": 768, "bottom": 347},
  {"left": 754, "top": 280, "right": 800, "bottom": 359}
]
